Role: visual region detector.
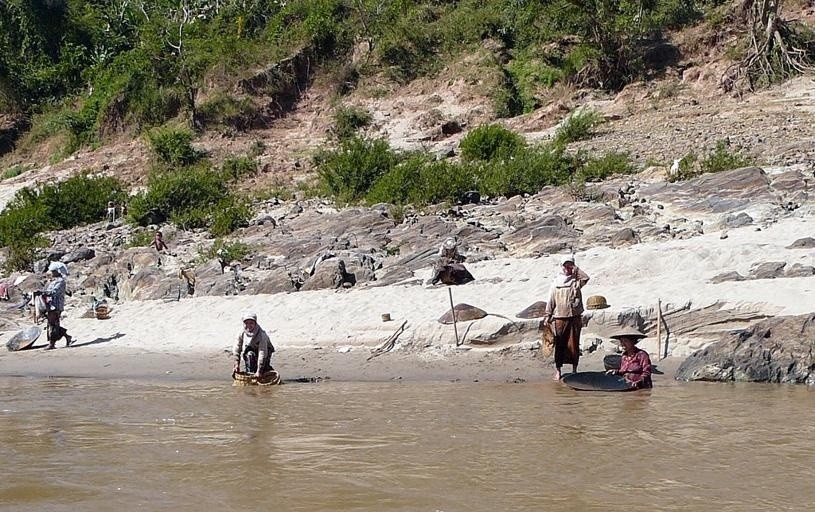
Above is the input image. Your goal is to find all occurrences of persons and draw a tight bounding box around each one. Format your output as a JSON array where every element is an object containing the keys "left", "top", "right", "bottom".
[
  {"left": 543, "top": 256, "right": 592, "bottom": 381},
  {"left": 32, "top": 262, "right": 72, "bottom": 352},
  {"left": 605, "top": 327, "right": 653, "bottom": 389},
  {"left": 227, "top": 310, "right": 276, "bottom": 380}
]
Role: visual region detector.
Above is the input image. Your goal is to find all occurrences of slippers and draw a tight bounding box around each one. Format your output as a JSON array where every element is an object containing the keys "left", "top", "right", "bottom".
[{"left": 65, "top": 340, "right": 77, "bottom": 348}]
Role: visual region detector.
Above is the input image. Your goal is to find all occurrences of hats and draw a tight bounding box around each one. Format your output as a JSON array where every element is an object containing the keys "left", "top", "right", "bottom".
[
  {"left": 241, "top": 313, "right": 257, "bottom": 321},
  {"left": 542, "top": 324, "right": 555, "bottom": 358},
  {"left": 609, "top": 326, "right": 647, "bottom": 339},
  {"left": 560, "top": 257, "right": 575, "bottom": 266}
]
